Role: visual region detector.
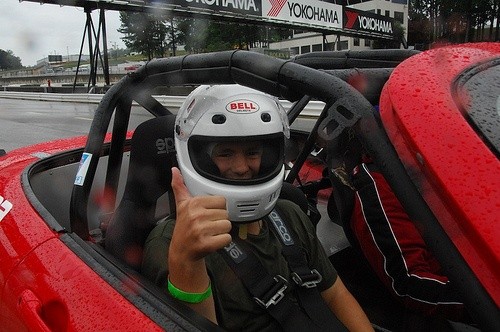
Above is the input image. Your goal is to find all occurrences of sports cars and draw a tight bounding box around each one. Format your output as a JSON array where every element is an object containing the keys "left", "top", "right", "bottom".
[{"left": 0, "top": 42, "right": 500, "bottom": 332}]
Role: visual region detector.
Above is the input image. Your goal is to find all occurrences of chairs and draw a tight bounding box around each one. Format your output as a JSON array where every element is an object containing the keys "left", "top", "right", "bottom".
[{"left": 105, "top": 113, "right": 177, "bottom": 273}]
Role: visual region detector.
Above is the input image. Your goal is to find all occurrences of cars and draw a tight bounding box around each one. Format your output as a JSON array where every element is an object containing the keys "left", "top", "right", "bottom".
[
  {"left": 123, "top": 64, "right": 142, "bottom": 71},
  {"left": 46, "top": 64, "right": 90, "bottom": 73}
]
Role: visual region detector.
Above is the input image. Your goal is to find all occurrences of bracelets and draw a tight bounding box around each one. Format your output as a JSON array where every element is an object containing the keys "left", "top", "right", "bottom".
[{"left": 167, "top": 279, "right": 212, "bottom": 304}]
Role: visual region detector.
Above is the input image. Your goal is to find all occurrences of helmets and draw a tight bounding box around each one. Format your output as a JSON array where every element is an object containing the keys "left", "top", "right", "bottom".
[{"left": 174, "top": 80, "right": 291, "bottom": 223}]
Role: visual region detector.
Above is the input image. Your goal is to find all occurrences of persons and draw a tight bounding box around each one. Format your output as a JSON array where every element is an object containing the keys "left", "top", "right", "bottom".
[
  {"left": 350, "top": 157, "right": 469, "bottom": 320},
  {"left": 147, "top": 84, "right": 375, "bottom": 332}
]
